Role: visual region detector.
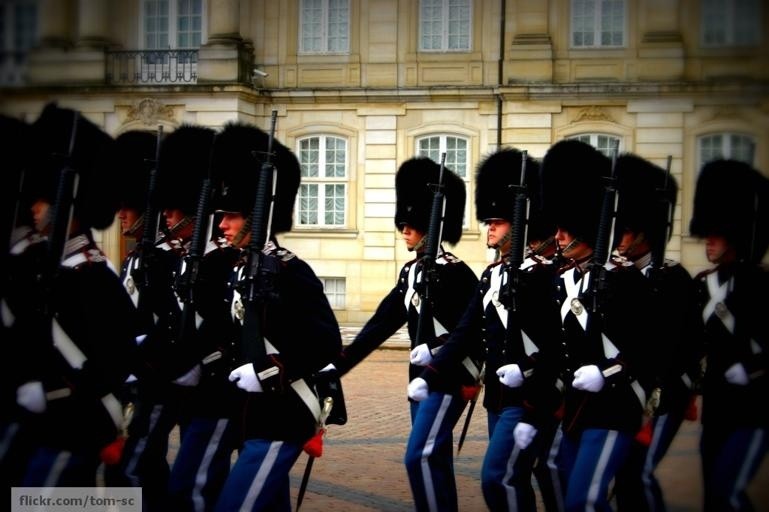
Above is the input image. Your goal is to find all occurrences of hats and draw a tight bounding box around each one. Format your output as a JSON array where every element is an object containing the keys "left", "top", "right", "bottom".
[
  {"left": 3, "top": 100, "right": 299, "bottom": 234},
  {"left": 688, "top": 157, "right": 768, "bottom": 258},
  {"left": 393, "top": 154, "right": 466, "bottom": 244},
  {"left": 473, "top": 140, "right": 677, "bottom": 264}
]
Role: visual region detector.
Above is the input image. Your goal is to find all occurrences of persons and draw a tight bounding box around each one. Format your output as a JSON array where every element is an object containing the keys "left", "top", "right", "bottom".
[
  {"left": 405, "top": 217, "right": 769, "bottom": 512},
  {"left": 341, "top": 220, "right": 484, "bottom": 511},
  {"left": 0, "top": 200, "right": 348, "bottom": 512}
]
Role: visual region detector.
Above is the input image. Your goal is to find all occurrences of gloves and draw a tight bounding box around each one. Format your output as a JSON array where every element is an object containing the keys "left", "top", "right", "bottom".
[
  {"left": 512, "top": 422, "right": 538, "bottom": 448},
  {"left": 495, "top": 363, "right": 524, "bottom": 390},
  {"left": 405, "top": 377, "right": 430, "bottom": 403},
  {"left": 227, "top": 362, "right": 261, "bottom": 394},
  {"left": 724, "top": 364, "right": 747, "bottom": 387},
  {"left": 571, "top": 365, "right": 605, "bottom": 394},
  {"left": 16, "top": 380, "right": 48, "bottom": 412},
  {"left": 405, "top": 342, "right": 434, "bottom": 368}
]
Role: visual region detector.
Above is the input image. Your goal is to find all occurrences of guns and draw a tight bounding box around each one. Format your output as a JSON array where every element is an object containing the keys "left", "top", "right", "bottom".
[
  {"left": 646, "top": 153, "right": 673, "bottom": 394},
  {"left": 130, "top": 123, "right": 165, "bottom": 335},
  {"left": 729, "top": 182, "right": 759, "bottom": 365},
  {"left": 500, "top": 149, "right": 530, "bottom": 365},
  {"left": 234, "top": 109, "right": 280, "bottom": 370},
  {"left": 580, "top": 138, "right": 621, "bottom": 367},
  {"left": 170, "top": 133, "right": 220, "bottom": 376},
  {"left": 412, "top": 151, "right": 447, "bottom": 344},
  {"left": 25, "top": 106, "right": 81, "bottom": 381}
]
